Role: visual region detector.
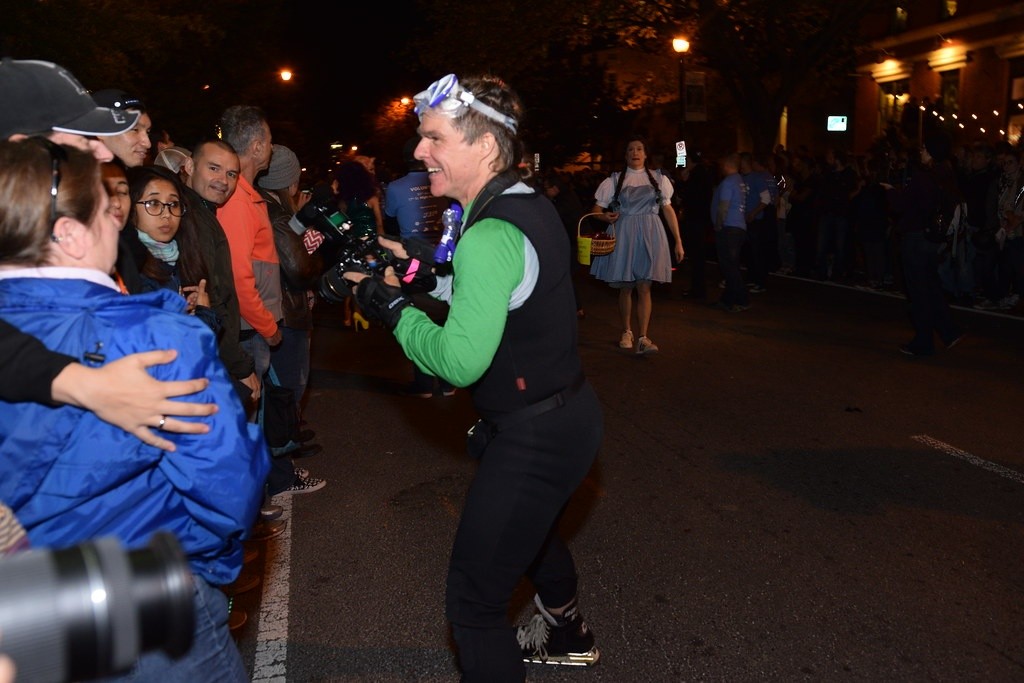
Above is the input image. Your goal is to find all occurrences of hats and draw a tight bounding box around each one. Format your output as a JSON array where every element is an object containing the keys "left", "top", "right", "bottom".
[
  {"left": 260, "top": 144, "right": 301, "bottom": 188},
  {"left": 1, "top": 59, "right": 142, "bottom": 136},
  {"left": 154, "top": 147, "right": 192, "bottom": 173}
]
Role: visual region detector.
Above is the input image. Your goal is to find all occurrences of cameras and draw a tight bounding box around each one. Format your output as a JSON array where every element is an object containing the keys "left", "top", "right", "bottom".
[
  {"left": 317, "top": 204, "right": 395, "bottom": 303},
  {"left": 1, "top": 532, "right": 198, "bottom": 683}
]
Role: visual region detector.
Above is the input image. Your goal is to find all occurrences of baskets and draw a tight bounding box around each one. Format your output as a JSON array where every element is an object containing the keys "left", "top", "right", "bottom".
[{"left": 578, "top": 213, "right": 615, "bottom": 256}]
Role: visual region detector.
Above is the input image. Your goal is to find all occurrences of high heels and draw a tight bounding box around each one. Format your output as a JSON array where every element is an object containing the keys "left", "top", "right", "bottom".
[{"left": 354, "top": 312, "right": 369, "bottom": 332}]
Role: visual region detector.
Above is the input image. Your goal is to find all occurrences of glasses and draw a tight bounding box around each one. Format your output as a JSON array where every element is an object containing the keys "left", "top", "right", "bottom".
[
  {"left": 136, "top": 199, "right": 187, "bottom": 217},
  {"left": 414, "top": 74, "right": 457, "bottom": 114},
  {"left": 1002, "top": 158, "right": 1018, "bottom": 166}
]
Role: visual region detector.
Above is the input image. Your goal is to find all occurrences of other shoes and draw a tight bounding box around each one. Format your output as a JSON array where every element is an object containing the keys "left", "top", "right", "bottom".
[
  {"left": 245, "top": 543, "right": 259, "bottom": 565},
  {"left": 259, "top": 506, "right": 283, "bottom": 519},
  {"left": 286, "top": 479, "right": 327, "bottom": 493},
  {"left": 218, "top": 601, "right": 248, "bottom": 629},
  {"left": 972, "top": 299, "right": 999, "bottom": 309},
  {"left": 251, "top": 519, "right": 286, "bottom": 541},
  {"left": 897, "top": 343, "right": 935, "bottom": 359},
  {"left": 294, "top": 467, "right": 309, "bottom": 477},
  {"left": 748, "top": 284, "right": 766, "bottom": 293},
  {"left": 234, "top": 566, "right": 262, "bottom": 594},
  {"left": 944, "top": 338, "right": 961, "bottom": 351},
  {"left": 999, "top": 295, "right": 1020, "bottom": 309},
  {"left": 294, "top": 444, "right": 322, "bottom": 457},
  {"left": 296, "top": 429, "right": 314, "bottom": 442}
]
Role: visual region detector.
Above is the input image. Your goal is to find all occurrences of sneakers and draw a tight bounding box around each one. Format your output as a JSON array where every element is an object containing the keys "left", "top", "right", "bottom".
[
  {"left": 511, "top": 594, "right": 601, "bottom": 667},
  {"left": 620, "top": 330, "right": 633, "bottom": 348},
  {"left": 635, "top": 338, "right": 658, "bottom": 354},
  {"left": 413, "top": 376, "right": 460, "bottom": 400}
]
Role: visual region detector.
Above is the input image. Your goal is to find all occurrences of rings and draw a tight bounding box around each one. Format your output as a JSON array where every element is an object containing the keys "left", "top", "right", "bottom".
[{"left": 158, "top": 416, "right": 167, "bottom": 431}]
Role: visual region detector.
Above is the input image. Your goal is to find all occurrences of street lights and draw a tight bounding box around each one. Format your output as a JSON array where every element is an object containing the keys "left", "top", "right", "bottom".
[{"left": 672, "top": 33, "right": 690, "bottom": 143}]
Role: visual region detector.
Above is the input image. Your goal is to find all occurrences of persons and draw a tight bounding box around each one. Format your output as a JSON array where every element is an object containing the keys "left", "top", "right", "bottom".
[
  {"left": 531, "top": 168, "right": 624, "bottom": 281},
  {"left": 593, "top": 138, "right": 685, "bottom": 355},
  {"left": 345, "top": 76, "right": 603, "bottom": 683},
  {"left": 646, "top": 127, "right": 1024, "bottom": 358},
  {"left": 0, "top": 60, "right": 460, "bottom": 683}
]
